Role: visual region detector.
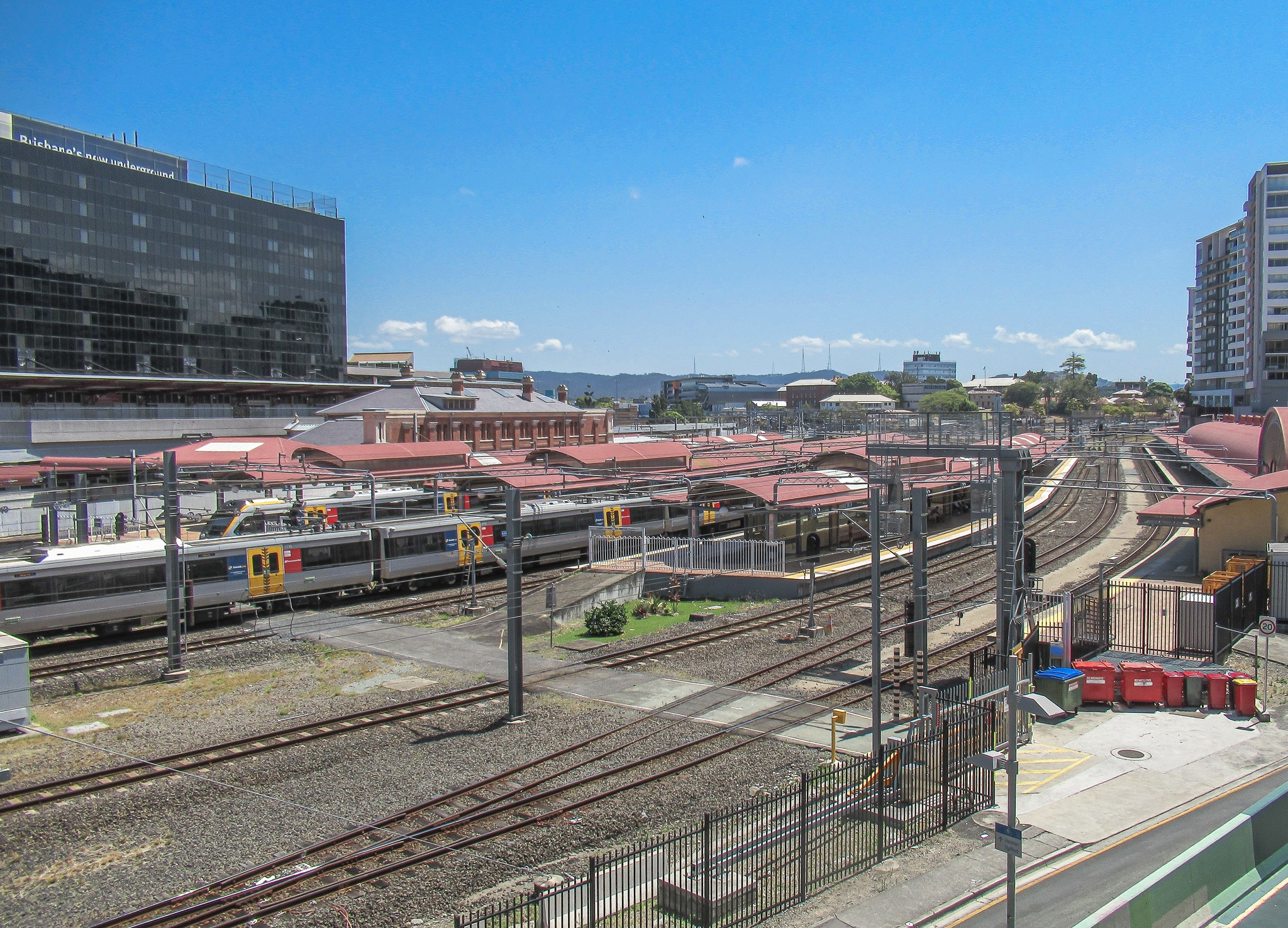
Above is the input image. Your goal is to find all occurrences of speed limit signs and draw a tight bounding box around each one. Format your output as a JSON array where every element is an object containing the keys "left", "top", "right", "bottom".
[{"left": 1258, "top": 616, "right": 1276, "bottom": 637}]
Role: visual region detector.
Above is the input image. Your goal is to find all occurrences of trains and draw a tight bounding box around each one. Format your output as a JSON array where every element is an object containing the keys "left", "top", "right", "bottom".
[
  {"left": 0, "top": 490, "right": 769, "bottom": 647},
  {"left": 745, "top": 482, "right": 974, "bottom": 558},
  {"left": 199, "top": 487, "right": 556, "bottom": 542}
]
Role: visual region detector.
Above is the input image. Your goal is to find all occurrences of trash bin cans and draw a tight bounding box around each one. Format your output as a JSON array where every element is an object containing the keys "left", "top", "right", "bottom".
[
  {"left": 1232, "top": 678, "right": 1257, "bottom": 716},
  {"left": 1117, "top": 660, "right": 1165, "bottom": 710},
  {"left": 1226, "top": 672, "right": 1252, "bottom": 710},
  {"left": 1163, "top": 671, "right": 1185, "bottom": 708},
  {"left": 1204, "top": 673, "right": 1229, "bottom": 711},
  {"left": 1034, "top": 665, "right": 1086, "bottom": 719},
  {"left": 1069, "top": 658, "right": 1117, "bottom": 707},
  {"left": 1182, "top": 670, "right": 1205, "bottom": 708}
]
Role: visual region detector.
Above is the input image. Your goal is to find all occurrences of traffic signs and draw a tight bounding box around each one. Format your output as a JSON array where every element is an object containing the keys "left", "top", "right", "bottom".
[{"left": 993, "top": 821, "right": 1022, "bottom": 858}]
